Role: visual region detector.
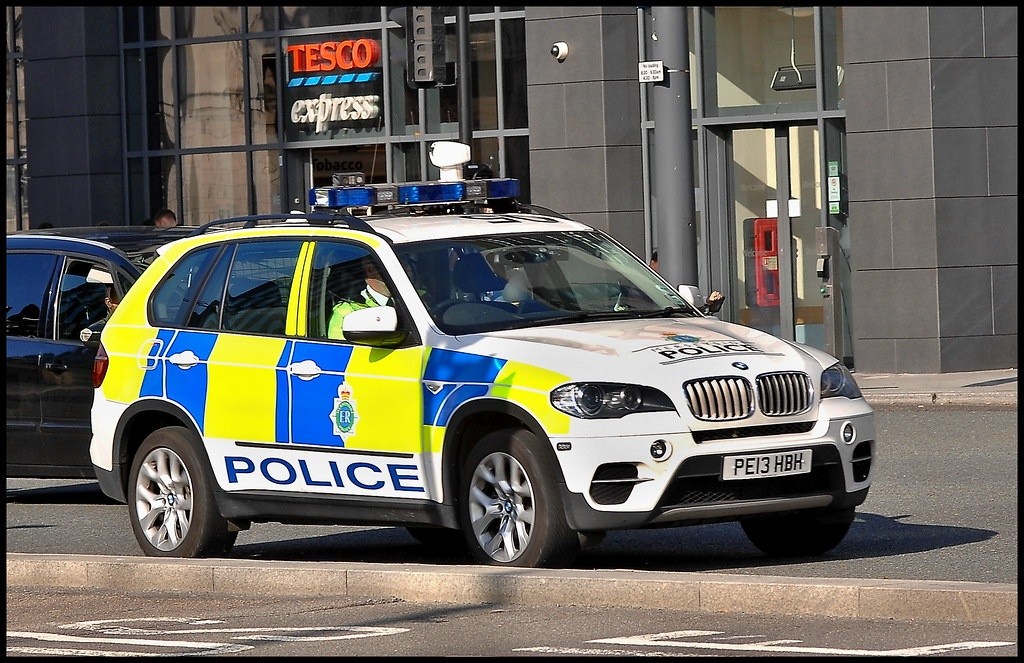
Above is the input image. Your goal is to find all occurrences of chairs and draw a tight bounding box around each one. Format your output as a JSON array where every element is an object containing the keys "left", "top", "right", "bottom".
[{"left": 452, "top": 253, "right": 507, "bottom": 303}]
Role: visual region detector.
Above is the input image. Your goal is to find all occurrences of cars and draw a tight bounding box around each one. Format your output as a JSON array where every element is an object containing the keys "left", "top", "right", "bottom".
[
  {"left": 88, "top": 141, "right": 877, "bottom": 569},
  {"left": 6, "top": 222, "right": 228, "bottom": 480}
]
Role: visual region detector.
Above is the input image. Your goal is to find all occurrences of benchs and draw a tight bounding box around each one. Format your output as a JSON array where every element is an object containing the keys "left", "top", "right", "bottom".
[{"left": 221, "top": 279, "right": 287, "bottom": 336}]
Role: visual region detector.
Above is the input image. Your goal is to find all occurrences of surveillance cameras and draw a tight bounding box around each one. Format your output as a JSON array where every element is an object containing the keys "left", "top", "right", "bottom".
[{"left": 550, "top": 42, "right": 568, "bottom": 59}]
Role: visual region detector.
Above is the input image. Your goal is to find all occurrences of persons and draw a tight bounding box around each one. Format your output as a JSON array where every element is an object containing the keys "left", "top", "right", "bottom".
[
  {"left": 328, "top": 256, "right": 433, "bottom": 348},
  {"left": 156, "top": 209, "right": 179, "bottom": 228},
  {"left": 89, "top": 283, "right": 122, "bottom": 330},
  {"left": 494, "top": 250, "right": 559, "bottom": 314}
]
